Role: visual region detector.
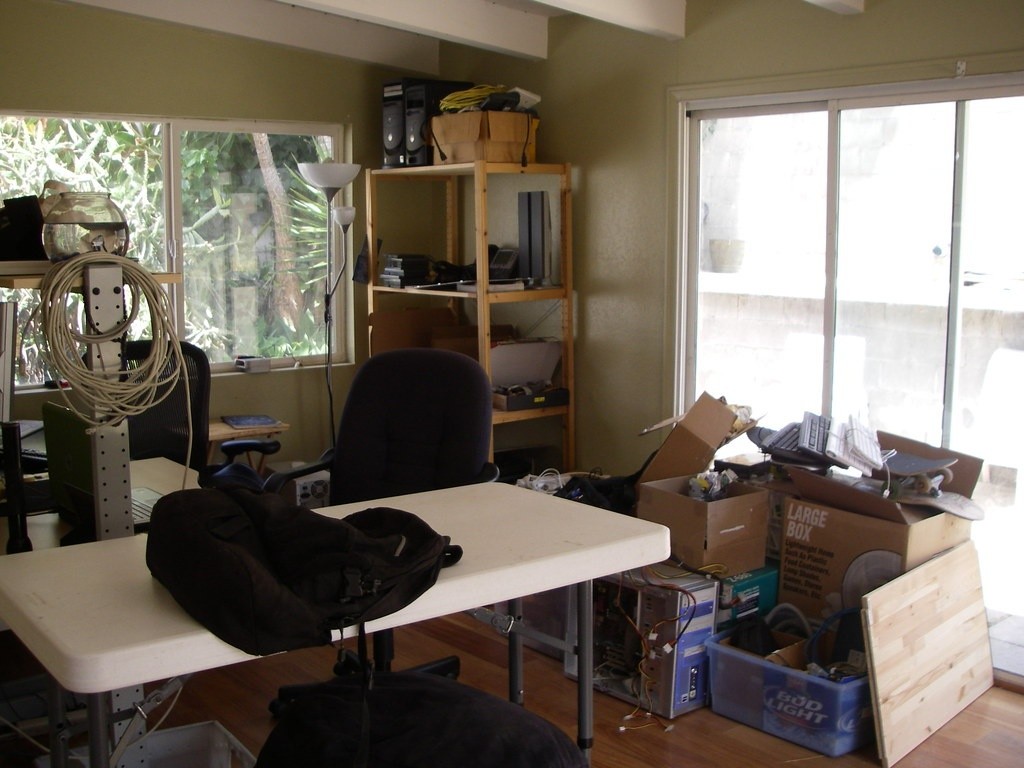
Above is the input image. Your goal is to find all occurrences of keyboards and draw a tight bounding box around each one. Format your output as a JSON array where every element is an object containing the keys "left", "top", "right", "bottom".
[
  {"left": 759, "top": 410, "right": 884, "bottom": 476},
  {"left": 21, "top": 448, "right": 47, "bottom": 467}
]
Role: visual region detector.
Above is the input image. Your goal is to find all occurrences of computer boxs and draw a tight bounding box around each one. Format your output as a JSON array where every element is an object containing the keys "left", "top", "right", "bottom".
[
  {"left": 563, "top": 563, "right": 718, "bottom": 718},
  {"left": 380, "top": 77, "right": 475, "bottom": 170},
  {"left": 260, "top": 459, "right": 330, "bottom": 509}
]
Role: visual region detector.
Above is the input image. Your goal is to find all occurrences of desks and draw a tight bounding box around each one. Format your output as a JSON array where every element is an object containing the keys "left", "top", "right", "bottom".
[
  {"left": 202, "top": 419, "right": 292, "bottom": 478},
  {"left": 0, "top": 479, "right": 669, "bottom": 768}
]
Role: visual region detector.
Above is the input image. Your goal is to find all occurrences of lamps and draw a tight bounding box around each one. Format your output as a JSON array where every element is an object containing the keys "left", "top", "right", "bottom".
[{"left": 297, "top": 162, "right": 362, "bottom": 460}]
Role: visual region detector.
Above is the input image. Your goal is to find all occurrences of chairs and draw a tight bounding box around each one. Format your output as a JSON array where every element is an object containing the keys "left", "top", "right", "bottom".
[
  {"left": 267, "top": 347, "right": 498, "bottom": 713},
  {"left": 73, "top": 339, "right": 210, "bottom": 471}
]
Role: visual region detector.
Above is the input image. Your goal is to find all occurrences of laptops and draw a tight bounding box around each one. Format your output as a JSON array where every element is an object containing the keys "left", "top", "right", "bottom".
[{"left": 42, "top": 400, "right": 166, "bottom": 533}]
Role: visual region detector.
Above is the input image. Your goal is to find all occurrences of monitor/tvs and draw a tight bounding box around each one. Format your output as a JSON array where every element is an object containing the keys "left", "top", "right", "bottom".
[{"left": 0, "top": 301, "right": 18, "bottom": 451}]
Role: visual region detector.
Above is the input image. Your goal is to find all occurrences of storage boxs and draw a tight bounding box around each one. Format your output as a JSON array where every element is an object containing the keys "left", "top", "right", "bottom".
[
  {"left": 638, "top": 392, "right": 983, "bottom": 759},
  {"left": 491, "top": 585, "right": 567, "bottom": 664},
  {"left": 33, "top": 718, "right": 262, "bottom": 768},
  {"left": 430, "top": 110, "right": 541, "bottom": 166}
]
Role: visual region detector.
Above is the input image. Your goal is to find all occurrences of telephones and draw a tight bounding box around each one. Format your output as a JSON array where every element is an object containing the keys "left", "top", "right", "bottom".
[{"left": 471, "top": 244, "right": 520, "bottom": 284}]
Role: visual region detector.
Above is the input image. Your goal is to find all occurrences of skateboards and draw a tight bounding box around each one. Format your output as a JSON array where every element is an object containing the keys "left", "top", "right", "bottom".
[{"left": 745, "top": 426, "right": 959, "bottom": 495}]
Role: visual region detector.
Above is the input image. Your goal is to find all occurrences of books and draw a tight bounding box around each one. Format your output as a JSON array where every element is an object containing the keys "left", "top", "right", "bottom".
[{"left": 222, "top": 413, "right": 284, "bottom": 428}]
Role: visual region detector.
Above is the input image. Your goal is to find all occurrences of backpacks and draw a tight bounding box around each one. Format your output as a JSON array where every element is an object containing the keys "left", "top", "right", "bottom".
[{"left": 146, "top": 484, "right": 463, "bottom": 656}]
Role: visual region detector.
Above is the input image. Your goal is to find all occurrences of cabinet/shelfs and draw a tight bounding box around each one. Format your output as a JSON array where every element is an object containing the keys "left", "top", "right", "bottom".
[{"left": 364, "top": 158, "right": 577, "bottom": 482}]
